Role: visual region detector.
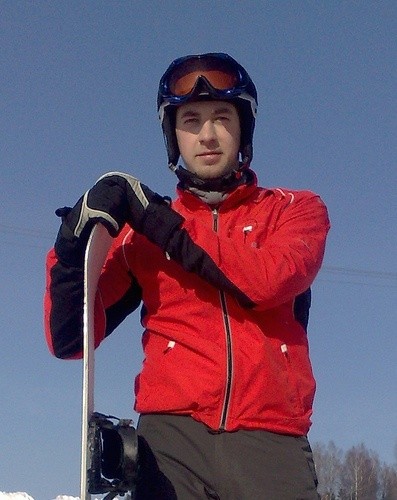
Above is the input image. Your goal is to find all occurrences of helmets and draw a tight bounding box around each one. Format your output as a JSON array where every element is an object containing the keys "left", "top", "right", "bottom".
[{"left": 157, "top": 53, "right": 258, "bottom": 111}]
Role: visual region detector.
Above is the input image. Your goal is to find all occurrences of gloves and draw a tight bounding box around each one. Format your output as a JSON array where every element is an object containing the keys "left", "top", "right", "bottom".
[
  {"left": 128, "top": 173, "right": 185, "bottom": 248},
  {"left": 54, "top": 172, "right": 127, "bottom": 260}
]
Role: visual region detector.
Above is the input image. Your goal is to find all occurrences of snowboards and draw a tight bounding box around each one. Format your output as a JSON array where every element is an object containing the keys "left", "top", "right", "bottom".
[{"left": 80, "top": 222, "right": 138, "bottom": 500}]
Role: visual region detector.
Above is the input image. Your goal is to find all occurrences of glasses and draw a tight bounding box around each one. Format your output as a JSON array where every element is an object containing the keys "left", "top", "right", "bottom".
[{"left": 169, "top": 57, "right": 238, "bottom": 96}]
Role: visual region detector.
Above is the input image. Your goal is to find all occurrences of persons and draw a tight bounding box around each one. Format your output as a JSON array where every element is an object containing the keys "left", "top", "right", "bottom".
[{"left": 43, "top": 52, "right": 331, "bottom": 500}]
